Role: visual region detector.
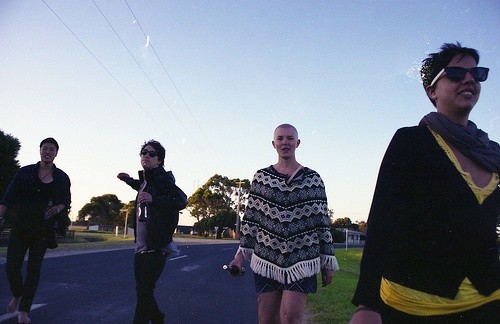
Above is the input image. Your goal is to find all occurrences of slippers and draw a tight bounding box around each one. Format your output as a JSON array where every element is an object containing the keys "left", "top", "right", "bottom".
[
  {"left": 18, "top": 310, "right": 31, "bottom": 324},
  {"left": 7, "top": 295, "right": 21, "bottom": 313}
]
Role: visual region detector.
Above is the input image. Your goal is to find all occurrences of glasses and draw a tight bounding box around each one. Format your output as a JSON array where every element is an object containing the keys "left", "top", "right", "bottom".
[
  {"left": 141, "top": 149, "right": 159, "bottom": 157},
  {"left": 430, "top": 67, "right": 489, "bottom": 86}
]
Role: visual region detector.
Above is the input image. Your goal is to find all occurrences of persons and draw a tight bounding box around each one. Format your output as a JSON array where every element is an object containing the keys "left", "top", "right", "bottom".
[
  {"left": 0, "top": 138, "right": 72, "bottom": 324},
  {"left": 351, "top": 43, "right": 500, "bottom": 324},
  {"left": 228, "top": 124, "right": 341, "bottom": 324},
  {"left": 117, "top": 140, "right": 188, "bottom": 324}
]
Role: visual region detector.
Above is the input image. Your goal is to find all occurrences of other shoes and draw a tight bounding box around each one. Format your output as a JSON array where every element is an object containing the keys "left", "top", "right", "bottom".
[{"left": 151, "top": 313, "right": 165, "bottom": 324}]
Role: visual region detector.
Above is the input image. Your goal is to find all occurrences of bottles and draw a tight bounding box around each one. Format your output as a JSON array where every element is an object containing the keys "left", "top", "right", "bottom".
[
  {"left": 137, "top": 201, "right": 147, "bottom": 221},
  {"left": 222, "top": 264, "right": 246, "bottom": 277}
]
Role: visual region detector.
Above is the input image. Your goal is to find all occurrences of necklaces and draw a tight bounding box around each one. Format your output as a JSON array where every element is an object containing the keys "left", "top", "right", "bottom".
[{"left": 40, "top": 167, "right": 53, "bottom": 180}]
